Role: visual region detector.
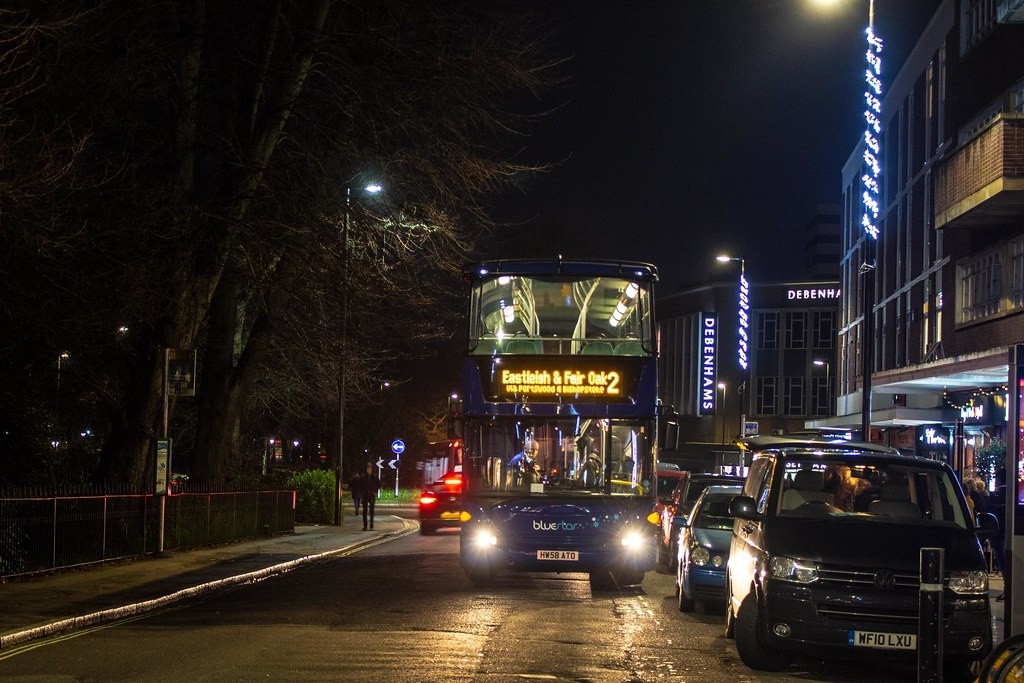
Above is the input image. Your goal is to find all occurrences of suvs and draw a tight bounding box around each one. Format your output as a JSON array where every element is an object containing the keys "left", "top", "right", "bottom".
[
  {"left": 723, "top": 434, "right": 1000, "bottom": 677},
  {"left": 658, "top": 470, "right": 747, "bottom": 576}
]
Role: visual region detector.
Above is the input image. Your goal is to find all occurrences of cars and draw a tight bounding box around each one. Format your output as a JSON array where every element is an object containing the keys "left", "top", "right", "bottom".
[
  {"left": 670, "top": 484, "right": 745, "bottom": 616},
  {"left": 420, "top": 465, "right": 464, "bottom": 535}
]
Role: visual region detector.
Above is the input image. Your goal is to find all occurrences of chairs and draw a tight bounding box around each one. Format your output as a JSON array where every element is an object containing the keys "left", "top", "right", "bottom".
[
  {"left": 870, "top": 480, "right": 923, "bottom": 519},
  {"left": 781, "top": 470, "right": 839, "bottom": 519}
]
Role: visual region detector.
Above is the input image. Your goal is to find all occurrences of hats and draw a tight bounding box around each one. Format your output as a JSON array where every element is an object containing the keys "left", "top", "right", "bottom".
[{"left": 993, "top": 468, "right": 1007, "bottom": 479}]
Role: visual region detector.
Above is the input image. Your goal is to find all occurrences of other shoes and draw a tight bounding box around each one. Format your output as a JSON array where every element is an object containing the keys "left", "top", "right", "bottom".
[{"left": 996, "top": 592, "right": 1004, "bottom": 603}]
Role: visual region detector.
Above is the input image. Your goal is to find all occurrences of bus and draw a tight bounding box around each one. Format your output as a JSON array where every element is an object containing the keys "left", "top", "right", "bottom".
[{"left": 446, "top": 257, "right": 680, "bottom": 592}]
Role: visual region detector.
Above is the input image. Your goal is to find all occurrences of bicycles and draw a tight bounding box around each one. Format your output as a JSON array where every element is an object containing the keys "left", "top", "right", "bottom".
[{"left": 978, "top": 633, "right": 1024, "bottom": 683}]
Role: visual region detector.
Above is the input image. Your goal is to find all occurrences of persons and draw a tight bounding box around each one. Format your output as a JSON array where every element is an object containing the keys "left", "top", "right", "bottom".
[
  {"left": 962, "top": 468, "right": 1006, "bottom": 527},
  {"left": 348, "top": 463, "right": 383, "bottom": 530},
  {"left": 577, "top": 436, "right": 601, "bottom": 490},
  {"left": 509, "top": 436, "right": 539, "bottom": 470}
]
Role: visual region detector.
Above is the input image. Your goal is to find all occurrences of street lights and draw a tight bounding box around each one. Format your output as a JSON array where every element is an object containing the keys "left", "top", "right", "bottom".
[
  {"left": 333, "top": 184, "right": 383, "bottom": 526},
  {"left": 715, "top": 257, "right": 750, "bottom": 439},
  {"left": 448, "top": 392, "right": 459, "bottom": 440},
  {"left": 814, "top": 0, "right": 884, "bottom": 446}
]
